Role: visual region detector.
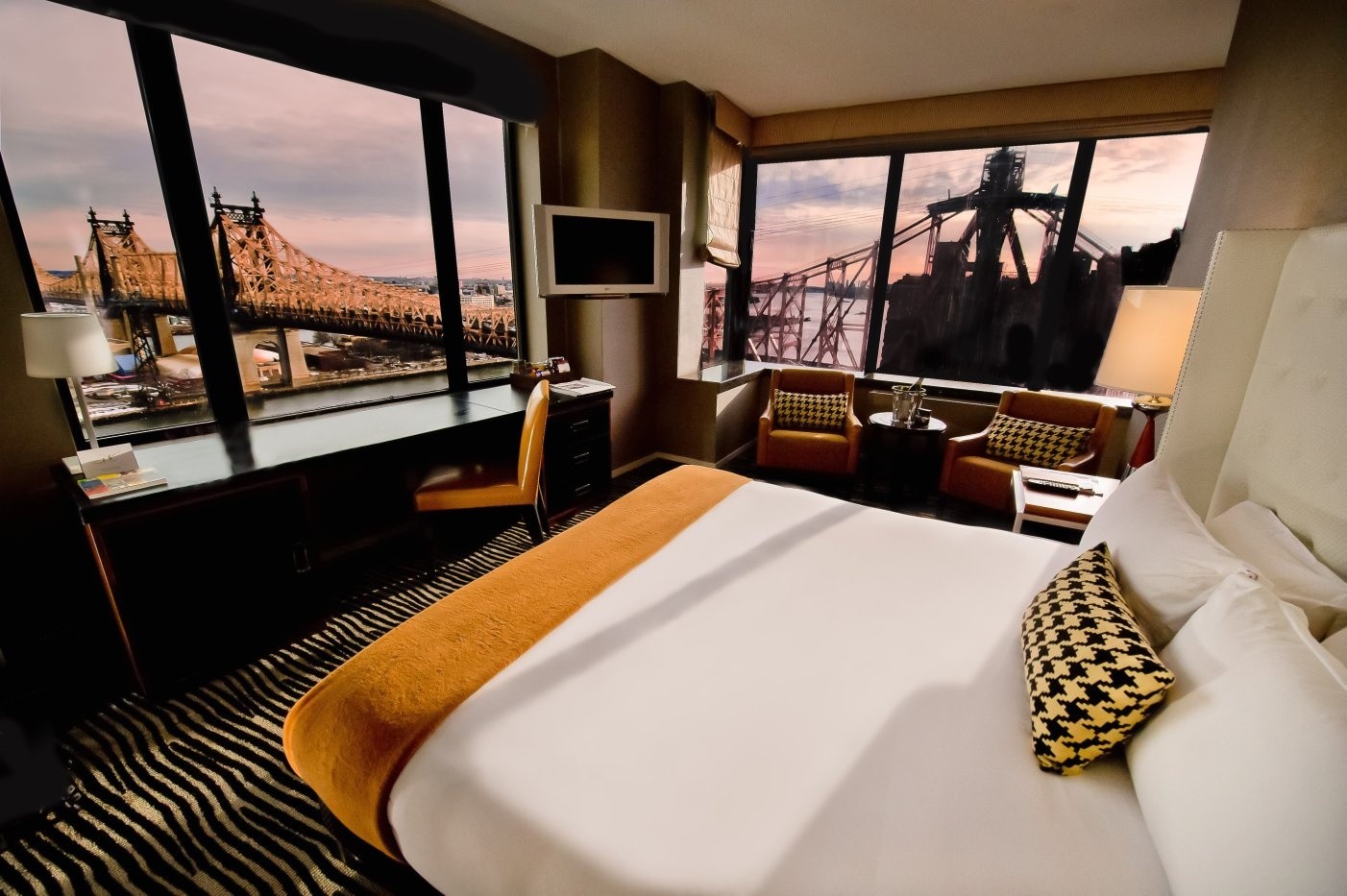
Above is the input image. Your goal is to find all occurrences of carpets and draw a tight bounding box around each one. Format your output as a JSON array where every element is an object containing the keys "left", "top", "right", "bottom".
[{"left": 0, "top": 498, "right": 610, "bottom": 896}]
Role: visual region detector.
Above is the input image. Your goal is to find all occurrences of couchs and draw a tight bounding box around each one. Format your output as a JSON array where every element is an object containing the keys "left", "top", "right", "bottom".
[
  {"left": 754, "top": 367, "right": 866, "bottom": 499},
  {"left": 940, "top": 389, "right": 1119, "bottom": 534}
]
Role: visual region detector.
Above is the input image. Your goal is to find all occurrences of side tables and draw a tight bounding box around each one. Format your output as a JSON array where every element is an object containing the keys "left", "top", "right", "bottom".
[{"left": 866, "top": 410, "right": 950, "bottom": 509}]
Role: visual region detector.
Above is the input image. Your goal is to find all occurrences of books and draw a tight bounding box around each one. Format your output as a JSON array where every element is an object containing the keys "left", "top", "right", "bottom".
[{"left": 76, "top": 467, "right": 167, "bottom": 500}]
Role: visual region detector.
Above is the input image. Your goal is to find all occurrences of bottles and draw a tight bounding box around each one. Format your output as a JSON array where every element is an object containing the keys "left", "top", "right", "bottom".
[{"left": 910, "top": 376, "right": 925, "bottom": 391}]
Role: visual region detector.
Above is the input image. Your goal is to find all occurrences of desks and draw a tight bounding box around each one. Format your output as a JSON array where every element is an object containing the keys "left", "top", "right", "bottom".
[
  {"left": 1010, "top": 464, "right": 1124, "bottom": 542},
  {"left": 59, "top": 358, "right": 619, "bottom": 704}
]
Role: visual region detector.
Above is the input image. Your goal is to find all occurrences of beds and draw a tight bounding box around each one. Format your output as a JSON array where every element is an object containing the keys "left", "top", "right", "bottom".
[{"left": 277, "top": 215, "right": 1347, "bottom": 896}]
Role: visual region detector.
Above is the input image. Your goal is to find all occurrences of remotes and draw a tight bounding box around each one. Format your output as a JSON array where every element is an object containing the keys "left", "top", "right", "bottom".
[{"left": 1028, "top": 478, "right": 1080, "bottom": 497}]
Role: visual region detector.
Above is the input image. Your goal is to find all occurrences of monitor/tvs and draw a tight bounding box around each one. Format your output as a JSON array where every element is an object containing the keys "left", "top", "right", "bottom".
[{"left": 532, "top": 204, "right": 671, "bottom": 295}]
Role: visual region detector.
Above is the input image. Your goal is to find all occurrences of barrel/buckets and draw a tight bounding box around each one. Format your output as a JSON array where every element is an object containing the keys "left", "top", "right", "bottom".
[{"left": 891, "top": 385, "right": 927, "bottom": 421}]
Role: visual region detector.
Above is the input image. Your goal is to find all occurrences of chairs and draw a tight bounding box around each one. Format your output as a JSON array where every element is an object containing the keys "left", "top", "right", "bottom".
[{"left": 409, "top": 378, "right": 557, "bottom": 569}]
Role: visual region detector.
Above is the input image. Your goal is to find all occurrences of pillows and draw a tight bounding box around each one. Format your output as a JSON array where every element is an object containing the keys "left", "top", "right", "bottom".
[
  {"left": 1209, "top": 497, "right": 1347, "bottom": 646},
  {"left": 1119, "top": 566, "right": 1347, "bottom": 896},
  {"left": 775, "top": 386, "right": 851, "bottom": 433},
  {"left": 1075, "top": 455, "right": 1269, "bottom": 660},
  {"left": 981, "top": 408, "right": 1096, "bottom": 473},
  {"left": 1019, "top": 539, "right": 1178, "bottom": 778}
]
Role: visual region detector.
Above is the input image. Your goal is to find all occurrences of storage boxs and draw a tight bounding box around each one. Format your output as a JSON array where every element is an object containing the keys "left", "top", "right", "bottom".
[{"left": 504, "top": 367, "right": 578, "bottom": 391}]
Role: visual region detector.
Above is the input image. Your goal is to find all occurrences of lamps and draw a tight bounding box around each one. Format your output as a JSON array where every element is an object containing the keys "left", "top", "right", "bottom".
[
  {"left": 18, "top": 307, "right": 132, "bottom": 473},
  {"left": 1092, "top": 282, "right": 1204, "bottom": 480}
]
